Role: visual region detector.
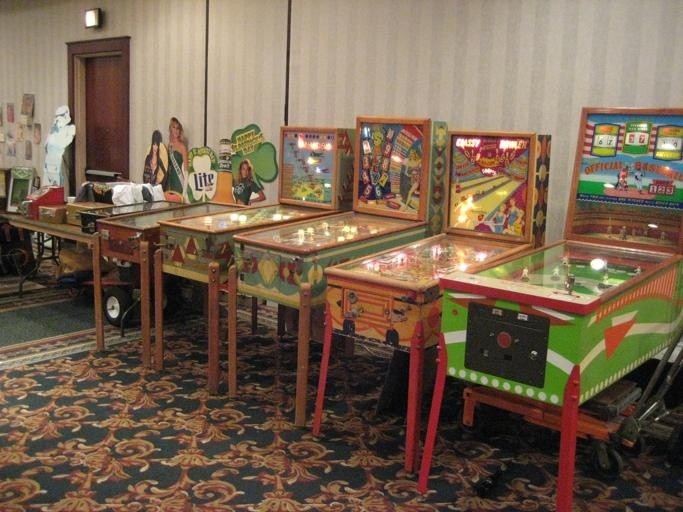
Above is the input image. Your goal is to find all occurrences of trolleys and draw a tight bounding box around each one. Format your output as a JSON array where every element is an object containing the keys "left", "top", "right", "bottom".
[{"left": 452, "top": 325, "right": 683, "bottom": 481}]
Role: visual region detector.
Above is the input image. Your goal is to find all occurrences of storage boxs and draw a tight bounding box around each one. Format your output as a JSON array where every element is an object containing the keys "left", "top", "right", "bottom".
[
  {"left": 65, "top": 202, "right": 114, "bottom": 227},
  {"left": 38, "top": 204, "right": 66, "bottom": 224}
]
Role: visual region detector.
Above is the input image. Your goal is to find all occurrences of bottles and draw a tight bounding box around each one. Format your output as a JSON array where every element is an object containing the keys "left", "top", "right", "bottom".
[{"left": 86, "top": 183, "right": 95, "bottom": 202}]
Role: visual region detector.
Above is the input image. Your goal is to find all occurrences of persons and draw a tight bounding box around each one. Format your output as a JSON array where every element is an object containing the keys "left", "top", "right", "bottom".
[
  {"left": 231, "top": 159, "right": 265, "bottom": 206},
  {"left": 141, "top": 129, "right": 165, "bottom": 194},
  {"left": 404, "top": 148, "right": 419, "bottom": 209},
  {"left": 166, "top": 116, "right": 189, "bottom": 195}
]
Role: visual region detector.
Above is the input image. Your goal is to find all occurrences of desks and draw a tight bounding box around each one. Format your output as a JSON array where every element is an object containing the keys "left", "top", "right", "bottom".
[{"left": 0, "top": 210, "right": 106, "bottom": 352}]
